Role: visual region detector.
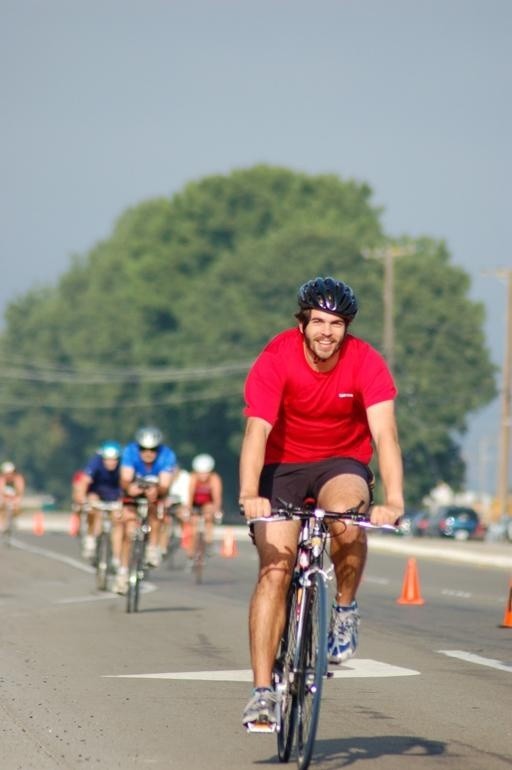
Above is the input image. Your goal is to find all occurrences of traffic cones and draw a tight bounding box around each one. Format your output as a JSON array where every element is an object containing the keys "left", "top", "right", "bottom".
[
  {"left": 389, "top": 555, "right": 423, "bottom": 612},
  {"left": 31, "top": 509, "right": 45, "bottom": 536},
  {"left": 221, "top": 529, "right": 240, "bottom": 559},
  {"left": 498, "top": 579, "right": 512, "bottom": 629}
]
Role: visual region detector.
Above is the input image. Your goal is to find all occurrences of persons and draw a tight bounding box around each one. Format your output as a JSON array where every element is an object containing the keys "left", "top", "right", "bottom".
[
  {"left": 237, "top": 275, "right": 405, "bottom": 727},
  {"left": 0, "top": 427, "right": 223, "bottom": 596}
]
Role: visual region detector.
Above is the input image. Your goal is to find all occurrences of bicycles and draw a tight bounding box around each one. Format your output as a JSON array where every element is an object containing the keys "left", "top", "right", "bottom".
[
  {"left": 0, "top": 497, "right": 20, "bottom": 545},
  {"left": 71, "top": 485, "right": 217, "bottom": 612},
  {"left": 243, "top": 497, "right": 403, "bottom": 770}
]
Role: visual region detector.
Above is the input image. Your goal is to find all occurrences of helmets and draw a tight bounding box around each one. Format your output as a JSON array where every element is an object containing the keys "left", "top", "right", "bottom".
[
  {"left": 133, "top": 425, "right": 164, "bottom": 449},
  {"left": 95, "top": 439, "right": 122, "bottom": 460},
  {"left": 191, "top": 452, "right": 215, "bottom": 474},
  {"left": 297, "top": 276, "right": 359, "bottom": 323},
  {"left": 0, "top": 462, "right": 16, "bottom": 475}
]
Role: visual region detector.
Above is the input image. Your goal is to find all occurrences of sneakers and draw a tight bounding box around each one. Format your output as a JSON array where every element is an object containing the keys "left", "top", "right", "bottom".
[
  {"left": 241, "top": 686, "right": 284, "bottom": 734},
  {"left": 81, "top": 534, "right": 168, "bottom": 596},
  {"left": 325, "top": 598, "right": 362, "bottom": 663}
]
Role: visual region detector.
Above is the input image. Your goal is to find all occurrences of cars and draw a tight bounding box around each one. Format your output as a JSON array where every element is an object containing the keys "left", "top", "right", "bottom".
[{"left": 396, "top": 505, "right": 487, "bottom": 540}]
